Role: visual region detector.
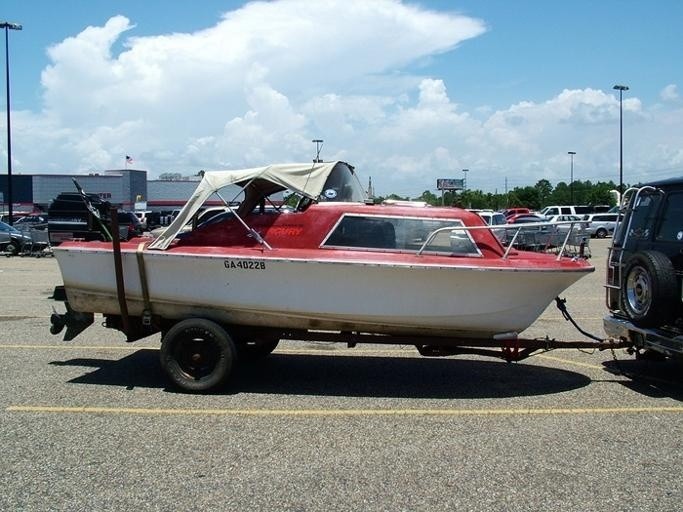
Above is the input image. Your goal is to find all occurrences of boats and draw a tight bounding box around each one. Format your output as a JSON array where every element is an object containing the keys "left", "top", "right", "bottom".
[{"left": 51, "top": 198, "right": 598, "bottom": 340}]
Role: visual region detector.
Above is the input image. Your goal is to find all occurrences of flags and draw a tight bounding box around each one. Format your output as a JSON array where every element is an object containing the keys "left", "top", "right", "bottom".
[{"left": 126, "top": 155, "right": 132, "bottom": 164}]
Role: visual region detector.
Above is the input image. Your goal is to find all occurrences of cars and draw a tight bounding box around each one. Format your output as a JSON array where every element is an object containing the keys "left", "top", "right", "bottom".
[
  {"left": 464, "top": 205, "right": 626, "bottom": 245},
  {"left": 0, "top": 191, "right": 192, "bottom": 254}
]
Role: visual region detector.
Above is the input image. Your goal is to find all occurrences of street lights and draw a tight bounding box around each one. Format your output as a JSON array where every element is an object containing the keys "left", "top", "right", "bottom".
[
  {"left": 312, "top": 139, "right": 320, "bottom": 162},
  {"left": 0, "top": 19, "right": 23, "bottom": 231},
  {"left": 462, "top": 169, "right": 469, "bottom": 191},
  {"left": 613, "top": 86, "right": 630, "bottom": 207},
  {"left": 568, "top": 151, "right": 575, "bottom": 203}
]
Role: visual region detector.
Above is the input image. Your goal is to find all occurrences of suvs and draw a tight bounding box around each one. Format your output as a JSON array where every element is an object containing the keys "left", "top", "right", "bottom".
[{"left": 601, "top": 176, "right": 682, "bottom": 362}]
